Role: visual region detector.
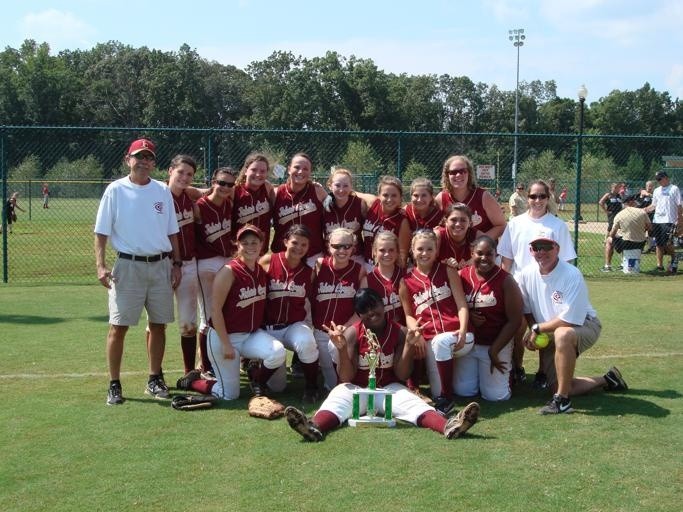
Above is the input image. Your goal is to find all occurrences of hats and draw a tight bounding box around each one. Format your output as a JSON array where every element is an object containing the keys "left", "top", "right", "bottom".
[
  {"left": 129, "top": 139, "right": 157, "bottom": 159},
  {"left": 236, "top": 224, "right": 264, "bottom": 240},
  {"left": 529, "top": 228, "right": 558, "bottom": 246}
]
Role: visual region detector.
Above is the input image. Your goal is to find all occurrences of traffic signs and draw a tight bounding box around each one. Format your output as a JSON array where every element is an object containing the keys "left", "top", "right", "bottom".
[{"left": 477, "top": 165, "right": 496, "bottom": 180}]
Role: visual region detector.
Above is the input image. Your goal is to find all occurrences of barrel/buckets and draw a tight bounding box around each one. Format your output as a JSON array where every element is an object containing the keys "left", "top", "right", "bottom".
[{"left": 623, "top": 249, "right": 642, "bottom": 274}]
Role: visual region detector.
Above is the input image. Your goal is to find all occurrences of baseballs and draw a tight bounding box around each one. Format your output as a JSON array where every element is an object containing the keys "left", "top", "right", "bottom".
[{"left": 536, "top": 334, "right": 549, "bottom": 347}]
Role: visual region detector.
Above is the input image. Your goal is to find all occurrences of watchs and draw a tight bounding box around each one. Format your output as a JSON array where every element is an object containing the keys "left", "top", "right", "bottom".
[{"left": 531, "top": 323, "right": 540, "bottom": 336}]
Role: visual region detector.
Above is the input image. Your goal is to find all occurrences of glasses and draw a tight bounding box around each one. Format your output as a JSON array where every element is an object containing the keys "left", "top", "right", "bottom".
[
  {"left": 528, "top": 193, "right": 546, "bottom": 201},
  {"left": 531, "top": 241, "right": 554, "bottom": 252},
  {"left": 217, "top": 181, "right": 235, "bottom": 188},
  {"left": 134, "top": 153, "right": 154, "bottom": 160},
  {"left": 516, "top": 188, "right": 524, "bottom": 190},
  {"left": 447, "top": 168, "right": 468, "bottom": 176},
  {"left": 330, "top": 243, "right": 353, "bottom": 250}
]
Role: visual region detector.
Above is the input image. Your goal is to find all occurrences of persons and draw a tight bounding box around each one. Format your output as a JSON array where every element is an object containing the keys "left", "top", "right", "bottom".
[
  {"left": 284, "top": 288, "right": 480, "bottom": 440},
  {"left": 167, "top": 151, "right": 524, "bottom": 402},
  {"left": 93, "top": 138, "right": 182, "bottom": 406},
  {"left": 517, "top": 226, "right": 629, "bottom": 415},
  {"left": 496, "top": 179, "right": 578, "bottom": 389},
  {"left": 598, "top": 170, "right": 683, "bottom": 275},
  {"left": 42, "top": 183, "right": 50, "bottom": 209},
  {"left": 558, "top": 189, "right": 567, "bottom": 211},
  {"left": 0, "top": 197, "right": 13, "bottom": 233},
  {"left": 10, "top": 192, "right": 26, "bottom": 228},
  {"left": 547, "top": 178, "right": 560, "bottom": 216}
]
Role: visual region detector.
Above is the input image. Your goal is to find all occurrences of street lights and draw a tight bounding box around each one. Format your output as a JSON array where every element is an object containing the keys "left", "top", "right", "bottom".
[
  {"left": 510, "top": 29, "right": 525, "bottom": 191},
  {"left": 573, "top": 84, "right": 587, "bottom": 220}
]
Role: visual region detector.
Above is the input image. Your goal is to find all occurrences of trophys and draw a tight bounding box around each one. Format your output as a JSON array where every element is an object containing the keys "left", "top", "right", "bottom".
[{"left": 347, "top": 328, "right": 396, "bottom": 428}]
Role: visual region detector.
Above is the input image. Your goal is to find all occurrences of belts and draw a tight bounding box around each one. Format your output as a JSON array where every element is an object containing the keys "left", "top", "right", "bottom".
[
  {"left": 118, "top": 252, "right": 171, "bottom": 262},
  {"left": 261, "top": 324, "right": 287, "bottom": 331}
]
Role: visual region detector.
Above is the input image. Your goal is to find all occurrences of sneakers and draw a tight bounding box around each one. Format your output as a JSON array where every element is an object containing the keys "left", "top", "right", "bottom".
[
  {"left": 531, "top": 371, "right": 551, "bottom": 389},
  {"left": 284, "top": 405, "right": 324, "bottom": 441},
  {"left": 289, "top": 360, "right": 304, "bottom": 378},
  {"left": 443, "top": 401, "right": 480, "bottom": 440},
  {"left": 538, "top": 393, "right": 574, "bottom": 415},
  {"left": 616, "top": 265, "right": 622, "bottom": 271},
  {"left": 598, "top": 267, "right": 612, "bottom": 272},
  {"left": 411, "top": 386, "right": 433, "bottom": 405},
  {"left": 106, "top": 383, "right": 123, "bottom": 405},
  {"left": 603, "top": 366, "right": 627, "bottom": 391},
  {"left": 510, "top": 367, "right": 527, "bottom": 386},
  {"left": 300, "top": 383, "right": 323, "bottom": 402},
  {"left": 145, "top": 359, "right": 272, "bottom": 399},
  {"left": 434, "top": 395, "right": 455, "bottom": 413}
]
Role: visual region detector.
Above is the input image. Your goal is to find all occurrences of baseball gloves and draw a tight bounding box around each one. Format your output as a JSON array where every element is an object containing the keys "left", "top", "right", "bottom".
[
  {"left": 249, "top": 394, "right": 284, "bottom": 419},
  {"left": 171, "top": 394, "right": 215, "bottom": 410}
]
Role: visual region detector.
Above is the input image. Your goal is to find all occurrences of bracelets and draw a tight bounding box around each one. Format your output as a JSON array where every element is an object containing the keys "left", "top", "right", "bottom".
[{"left": 175, "top": 262, "right": 182, "bottom": 268}]
